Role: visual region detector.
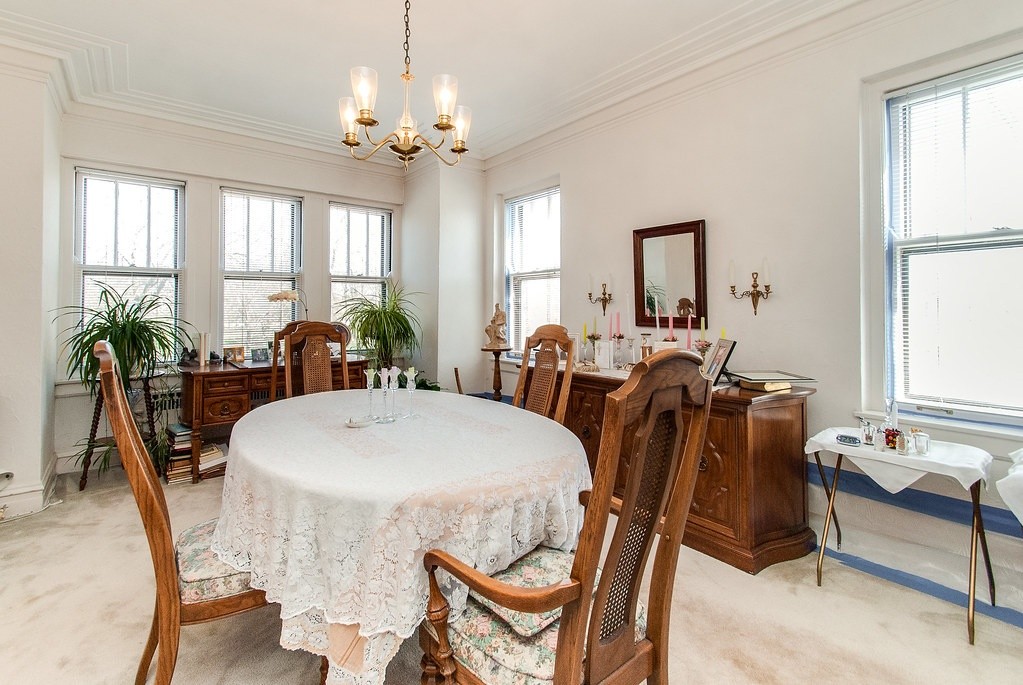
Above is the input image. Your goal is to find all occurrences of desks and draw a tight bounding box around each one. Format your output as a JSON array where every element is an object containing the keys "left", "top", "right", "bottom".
[
  {"left": 804, "top": 427, "right": 996, "bottom": 645},
  {"left": 79, "top": 369, "right": 165, "bottom": 491},
  {"left": 208, "top": 389, "right": 593, "bottom": 684}
]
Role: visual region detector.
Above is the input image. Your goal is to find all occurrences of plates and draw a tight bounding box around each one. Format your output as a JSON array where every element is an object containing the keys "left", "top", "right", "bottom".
[{"left": 345, "top": 418, "right": 371, "bottom": 426}]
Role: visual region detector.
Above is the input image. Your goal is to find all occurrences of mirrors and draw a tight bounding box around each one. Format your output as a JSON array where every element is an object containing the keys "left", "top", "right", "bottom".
[{"left": 632, "top": 219, "right": 709, "bottom": 330}]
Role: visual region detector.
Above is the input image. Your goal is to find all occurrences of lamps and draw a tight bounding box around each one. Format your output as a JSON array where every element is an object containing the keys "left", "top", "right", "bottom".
[{"left": 338, "top": 0, "right": 469, "bottom": 172}]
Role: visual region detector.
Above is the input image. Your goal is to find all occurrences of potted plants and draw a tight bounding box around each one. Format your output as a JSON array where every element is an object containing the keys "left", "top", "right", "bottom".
[{"left": 47, "top": 279, "right": 203, "bottom": 396}]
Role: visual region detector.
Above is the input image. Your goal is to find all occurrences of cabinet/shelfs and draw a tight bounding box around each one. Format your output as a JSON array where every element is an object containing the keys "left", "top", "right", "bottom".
[
  {"left": 517, "top": 362, "right": 817, "bottom": 576},
  {"left": 178, "top": 354, "right": 375, "bottom": 485}
]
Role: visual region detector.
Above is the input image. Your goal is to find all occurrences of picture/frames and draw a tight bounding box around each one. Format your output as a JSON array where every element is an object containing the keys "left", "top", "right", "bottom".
[
  {"left": 251, "top": 348, "right": 268, "bottom": 361},
  {"left": 704, "top": 339, "right": 736, "bottom": 387},
  {"left": 224, "top": 347, "right": 244, "bottom": 363}
]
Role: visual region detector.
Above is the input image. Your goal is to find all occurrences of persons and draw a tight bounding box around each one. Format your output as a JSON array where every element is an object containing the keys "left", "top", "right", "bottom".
[{"left": 484, "top": 302, "right": 505, "bottom": 346}]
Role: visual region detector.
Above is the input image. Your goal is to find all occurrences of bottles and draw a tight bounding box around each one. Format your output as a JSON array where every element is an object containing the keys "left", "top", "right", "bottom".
[
  {"left": 862, "top": 421, "right": 876, "bottom": 445},
  {"left": 873, "top": 429, "right": 886, "bottom": 452},
  {"left": 896, "top": 431, "right": 908, "bottom": 455}
]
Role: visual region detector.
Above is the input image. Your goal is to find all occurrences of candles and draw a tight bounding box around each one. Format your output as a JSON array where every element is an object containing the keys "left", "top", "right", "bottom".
[
  {"left": 581, "top": 265, "right": 771, "bottom": 354},
  {"left": 659, "top": 306, "right": 663, "bottom": 317},
  {"left": 364, "top": 365, "right": 415, "bottom": 386},
  {"left": 646, "top": 305, "right": 651, "bottom": 318}
]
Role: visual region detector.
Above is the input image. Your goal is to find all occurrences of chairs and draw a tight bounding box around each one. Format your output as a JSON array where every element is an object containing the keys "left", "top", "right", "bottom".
[
  {"left": 412, "top": 349, "right": 713, "bottom": 685},
  {"left": 267, "top": 320, "right": 349, "bottom": 402},
  {"left": 93, "top": 339, "right": 271, "bottom": 685}
]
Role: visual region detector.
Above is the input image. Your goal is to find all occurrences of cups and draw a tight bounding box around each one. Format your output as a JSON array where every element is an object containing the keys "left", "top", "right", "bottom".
[{"left": 913, "top": 433, "right": 929, "bottom": 456}]
